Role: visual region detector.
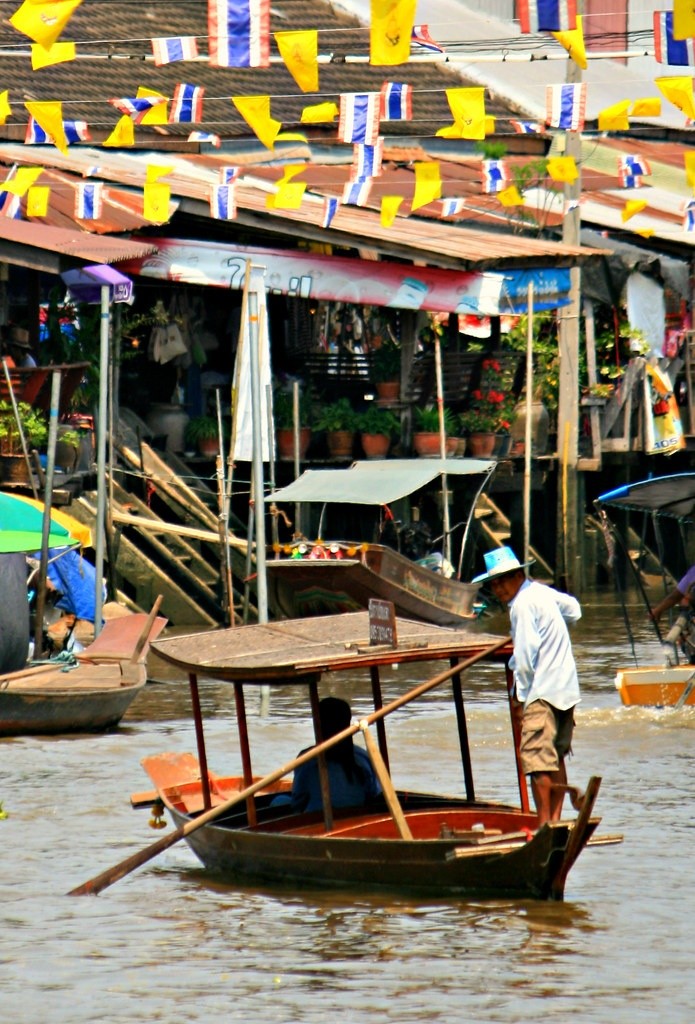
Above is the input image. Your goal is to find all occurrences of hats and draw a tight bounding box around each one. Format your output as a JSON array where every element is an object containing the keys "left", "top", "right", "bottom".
[
  {"left": 471, "top": 546, "right": 537, "bottom": 584},
  {"left": 4, "top": 327, "right": 32, "bottom": 348}
]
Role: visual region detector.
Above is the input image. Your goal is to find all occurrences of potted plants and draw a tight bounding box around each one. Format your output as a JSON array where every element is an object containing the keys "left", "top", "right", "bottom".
[
  {"left": 0, "top": 401, "right": 88, "bottom": 486},
  {"left": 182, "top": 416, "right": 220, "bottom": 456},
  {"left": 310, "top": 398, "right": 361, "bottom": 457},
  {"left": 273, "top": 374, "right": 317, "bottom": 461},
  {"left": 353, "top": 407, "right": 401, "bottom": 458},
  {"left": 500, "top": 310, "right": 652, "bottom": 456},
  {"left": 413, "top": 403, "right": 467, "bottom": 458},
  {"left": 126, "top": 300, "right": 206, "bottom": 452}
]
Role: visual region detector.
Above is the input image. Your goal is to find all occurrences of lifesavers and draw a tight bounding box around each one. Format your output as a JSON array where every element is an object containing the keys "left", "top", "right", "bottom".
[{"left": 310, "top": 546, "right": 341, "bottom": 559}]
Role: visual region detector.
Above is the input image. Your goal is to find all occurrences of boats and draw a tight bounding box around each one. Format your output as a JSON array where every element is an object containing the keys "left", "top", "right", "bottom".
[
  {"left": 131, "top": 600, "right": 622, "bottom": 900},
  {"left": 0, "top": 615, "right": 169, "bottom": 735},
  {"left": 593, "top": 468, "right": 695, "bottom": 709},
  {"left": 264, "top": 459, "right": 499, "bottom": 625}
]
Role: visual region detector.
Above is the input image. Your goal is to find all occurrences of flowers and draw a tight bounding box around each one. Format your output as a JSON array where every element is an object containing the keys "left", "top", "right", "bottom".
[{"left": 458, "top": 359, "right": 514, "bottom": 434}]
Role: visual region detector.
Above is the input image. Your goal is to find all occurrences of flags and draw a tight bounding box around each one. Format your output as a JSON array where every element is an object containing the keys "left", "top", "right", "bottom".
[{"left": 0, "top": 0, "right": 695, "bottom": 240}]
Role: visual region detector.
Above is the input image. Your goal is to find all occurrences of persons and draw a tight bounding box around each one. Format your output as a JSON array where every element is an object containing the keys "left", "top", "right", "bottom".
[
  {"left": 269, "top": 697, "right": 382, "bottom": 818},
  {"left": 647, "top": 566, "right": 695, "bottom": 624},
  {"left": 471, "top": 547, "right": 583, "bottom": 840}
]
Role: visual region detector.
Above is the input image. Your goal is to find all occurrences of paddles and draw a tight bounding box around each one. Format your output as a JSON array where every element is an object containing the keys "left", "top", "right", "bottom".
[
  {"left": 676, "top": 673, "right": 695, "bottom": 711},
  {"left": 68, "top": 633, "right": 513, "bottom": 898}
]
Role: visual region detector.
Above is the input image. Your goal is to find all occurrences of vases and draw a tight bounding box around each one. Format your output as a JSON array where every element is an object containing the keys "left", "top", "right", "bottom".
[
  {"left": 375, "top": 382, "right": 400, "bottom": 399},
  {"left": 468, "top": 434, "right": 512, "bottom": 458}
]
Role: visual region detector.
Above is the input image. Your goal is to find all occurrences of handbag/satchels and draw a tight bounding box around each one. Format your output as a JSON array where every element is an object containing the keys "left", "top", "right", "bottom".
[{"left": 148, "top": 317, "right": 190, "bottom": 365}]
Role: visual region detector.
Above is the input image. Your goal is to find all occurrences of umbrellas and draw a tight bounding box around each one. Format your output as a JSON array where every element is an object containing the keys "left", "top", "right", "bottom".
[{"left": 0, "top": 491, "right": 93, "bottom": 556}]
